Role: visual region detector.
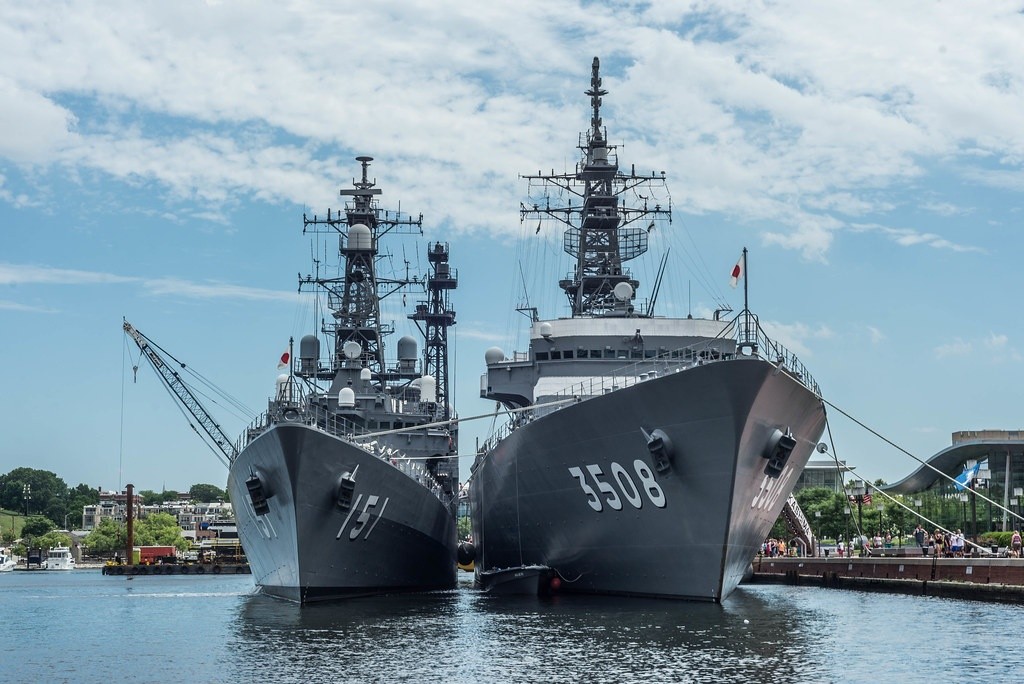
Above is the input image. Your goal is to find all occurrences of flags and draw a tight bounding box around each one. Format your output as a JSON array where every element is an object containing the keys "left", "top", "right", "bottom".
[
  {"left": 276, "top": 345, "right": 290, "bottom": 370},
  {"left": 729, "top": 254, "right": 745, "bottom": 289},
  {"left": 863, "top": 495, "right": 871, "bottom": 504}
]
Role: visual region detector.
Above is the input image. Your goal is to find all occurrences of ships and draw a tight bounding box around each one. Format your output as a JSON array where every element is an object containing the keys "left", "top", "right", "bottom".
[
  {"left": 125, "top": 153, "right": 461, "bottom": 603},
  {"left": 464, "top": 55, "right": 831, "bottom": 606}
]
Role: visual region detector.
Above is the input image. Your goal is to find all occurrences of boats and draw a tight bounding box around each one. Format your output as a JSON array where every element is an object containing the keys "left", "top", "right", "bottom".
[
  {"left": 0, "top": 555, "right": 17, "bottom": 572},
  {"left": 45, "top": 543, "right": 76, "bottom": 570},
  {"left": 14, "top": 555, "right": 47, "bottom": 570}
]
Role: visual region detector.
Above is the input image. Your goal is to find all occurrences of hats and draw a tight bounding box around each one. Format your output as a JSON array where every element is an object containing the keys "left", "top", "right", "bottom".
[{"left": 953, "top": 531, "right": 957, "bottom": 533}]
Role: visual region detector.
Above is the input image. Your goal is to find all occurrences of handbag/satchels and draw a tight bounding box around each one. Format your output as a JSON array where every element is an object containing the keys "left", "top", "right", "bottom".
[
  {"left": 874, "top": 537, "right": 878, "bottom": 548},
  {"left": 838, "top": 548, "right": 841, "bottom": 550}
]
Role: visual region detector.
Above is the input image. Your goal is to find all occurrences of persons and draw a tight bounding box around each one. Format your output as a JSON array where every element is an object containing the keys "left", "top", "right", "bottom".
[
  {"left": 1011, "top": 530, "right": 1022, "bottom": 558},
  {"left": 837, "top": 530, "right": 892, "bottom": 557},
  {"left": 913, "top": 525, "right": 965, "bottom": 558},
  {"left": 759, "top": 537, "right": 798, "bottom": 556}
]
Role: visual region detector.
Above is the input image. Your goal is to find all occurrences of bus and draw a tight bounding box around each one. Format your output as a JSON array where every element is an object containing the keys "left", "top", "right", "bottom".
[{"left": 81, "top": 547, "right": 98, "bottom": 561}]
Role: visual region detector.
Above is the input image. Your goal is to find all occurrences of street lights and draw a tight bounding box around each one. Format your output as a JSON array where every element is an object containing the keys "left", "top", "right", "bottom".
[
  {"left": 65, "top": 513, "right": 73, "bottom": 531},
  {"left": 961, "top": 494, "right": 970, "bottom": 553},
  {"left": 877, "top": 504, "right": 884, "bottom": 537},
  {"left": 815, "top": 510, "right": 822, "bottom": 556},
  {"left": 846, "top": 479, "right": 874, "bottom": 557},
  {"left": 843, "top": 507, "right": 850, "bottom": 558},
  {"left": 914, "top": 500, "right": 922, "bottom": 526},
  {"left": 23, "top": 484, "right": 33, "bottom": 516}
]
